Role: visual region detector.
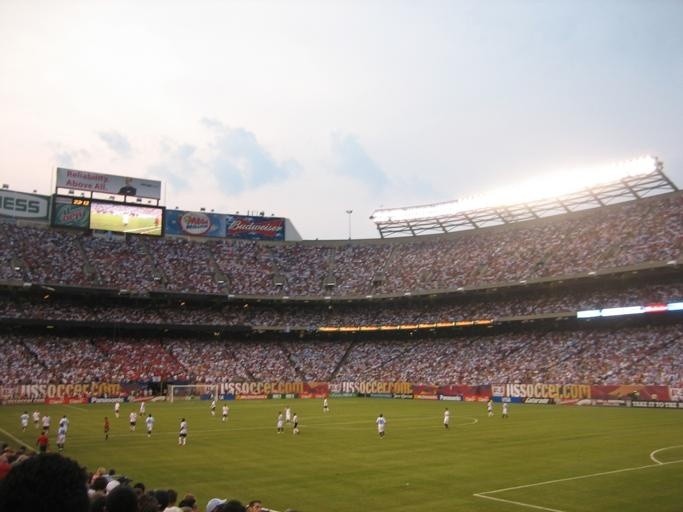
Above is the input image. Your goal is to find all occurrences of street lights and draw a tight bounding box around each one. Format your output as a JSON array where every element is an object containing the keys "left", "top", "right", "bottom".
[{"left": 346, "top": 210, "right": 353, "bottom": 240}]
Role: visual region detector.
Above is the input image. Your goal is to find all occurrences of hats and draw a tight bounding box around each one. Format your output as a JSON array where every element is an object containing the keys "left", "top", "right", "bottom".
[
  {"left": 206, "top": 498, "right": 227, "bottom": 512},
  {"left": 106, "top": 477, "right": 132, "bottom": 495}
]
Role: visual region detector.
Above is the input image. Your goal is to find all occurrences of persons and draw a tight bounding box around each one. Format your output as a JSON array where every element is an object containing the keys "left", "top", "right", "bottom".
[{"left": 117, "top": 178, "right": 136, "bottom": 196}]
